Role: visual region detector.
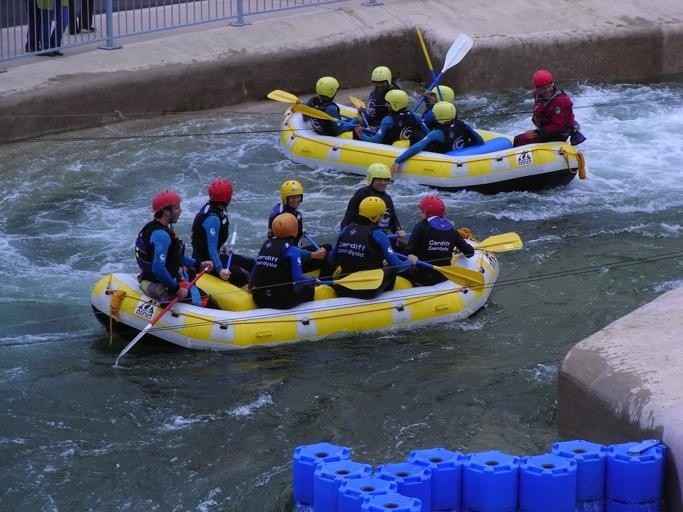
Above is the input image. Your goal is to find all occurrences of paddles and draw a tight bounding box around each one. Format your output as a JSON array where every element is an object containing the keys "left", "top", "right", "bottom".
[
  {"left": 266, "top": 89, "right": 357, "bottom": 121},
  {"left": 413, "top": 33, "right": 474, "bottom": 114},
  {"left": 311, "top": 269, "right": 385, "bottom": 291},
  {"left": 117, "top": 266, "right": 208, "bottom": 359},
  {"left": 227, "top": 221, "right": 238, "bottom": 271},
  {"left": 460, "top": 233, "right": 523, "bottom": 253},
  {"left": 294, "top": 104, "right": 378, "bottom": 133},
  {"left": 348, "top": 96, "right": 371, "bottom": 127},
  {"left": 415, "top": 25, "right": 444, "bottom": 101},
  {"left": 396, "top": 252, "right": 485, "bottom": 292}
]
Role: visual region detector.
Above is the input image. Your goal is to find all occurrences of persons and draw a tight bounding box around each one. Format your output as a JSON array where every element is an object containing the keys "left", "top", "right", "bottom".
[
  {"left": 513, "top": 69, "right": 575, "bottom": 148},
  {"left": 341, "top": 162, "right": 408, "bottom": 254},
  {"left": 268, "top": 179, "right": 336, "bottom": 285},
  {"left": 402, "top": 196, "right": 474, "bottom": 286},
  {"left": 191, "top": 177, "right": 257, "bottom": 288},
  {"left": 134, "top": 189, "right": 214, "bottom": 307},
  {"left": 302, "top": 66, "right": 484, "bottom": 177},
  {"left": 26, "top": 0, "right": 96, "bottom": 57},
  {"left": 327, "top": 195, "right": 419, "bottom": 299},
  {"left": 247, "top": 212, "right": 321, "bottom": 308}
]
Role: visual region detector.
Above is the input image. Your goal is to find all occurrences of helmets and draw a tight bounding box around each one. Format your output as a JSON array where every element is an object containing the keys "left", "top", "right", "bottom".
[
  {"left": 358, "top": 196, "right": 387, "bottom": 224},
  {"left": 281, "top": 179, "right": 303, "bottom": 206},
  {"left": 431, "top": 85, "right": 455, "bottom": 102},
  {"left": 366, "top": 163, "right": 392, "bottom": 186},
  {"left": 208, "top": 178, "right": 233, "bottom": 203},
  {"left": 151, "top": 191, "right": 182, "bottom": 213},
  {"left": 385, "top": 89, "right": 409, "bottom": 111},
  {"left": 271, "top": 213, "right": 300, "bottom": 238},
  {"left": 418, "top": 195, "right": 446, "bottom": 217},
  {"left": 432, "top": 101, "right": 456, "bottom": 124},
  {"left": 532, "top": 69, "right": 554, "bottom": 87},
  {"left": 371, "top": 65, "right": 392, "bottom": 85},
  {"left": 315, "top": 76, "right": 339, "bottom": 99}
]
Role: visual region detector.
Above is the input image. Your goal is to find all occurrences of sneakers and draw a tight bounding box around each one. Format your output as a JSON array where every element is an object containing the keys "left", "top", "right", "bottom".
[{"left": 25, "top": 24, "right": 96, "bottom": 56}]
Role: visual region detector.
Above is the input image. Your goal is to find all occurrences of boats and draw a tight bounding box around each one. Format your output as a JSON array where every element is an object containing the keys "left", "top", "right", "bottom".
[
  {"left": 89, "top": 234, "right": 499, "bottom": 352},
  {"left": 279, "top": 101, "right": 587, "bottom": 195}
]
